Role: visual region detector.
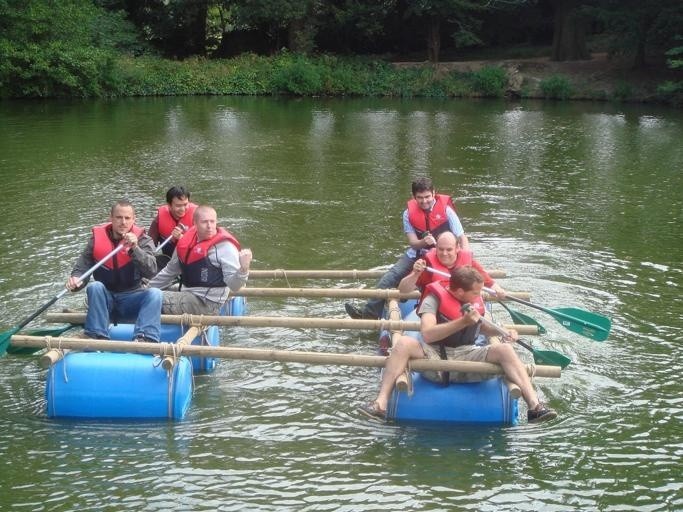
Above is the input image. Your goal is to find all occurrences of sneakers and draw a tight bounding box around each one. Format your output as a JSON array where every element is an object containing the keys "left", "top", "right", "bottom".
[
  {"left": 527, "top": 404, "right": 557, "bottom": 425},
  {"left": 356, "top": 400, "right": 388, "bottom": 424}
]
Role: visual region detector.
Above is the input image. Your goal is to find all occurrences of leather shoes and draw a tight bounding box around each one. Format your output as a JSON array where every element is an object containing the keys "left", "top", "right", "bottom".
[{"left": 344, "top": 302, "right": 362, "bottom": 319}]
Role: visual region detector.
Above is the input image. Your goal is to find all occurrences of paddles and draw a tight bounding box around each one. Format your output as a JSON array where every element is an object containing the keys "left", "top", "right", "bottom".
[
  {"left": 462, "top": 302, "right": 571, "bottom": 370},
  {"left": 421, "top": 266, "right": 610, "bottom": 342},
  {"left": 6, "top": 275, "right": 183, "bottom": 354},
  {"left": 498, "top": 301, "right": 546, "bottom": 334},
  {"left": 0, "top": 241, "right": 128, "bottom": 359}
]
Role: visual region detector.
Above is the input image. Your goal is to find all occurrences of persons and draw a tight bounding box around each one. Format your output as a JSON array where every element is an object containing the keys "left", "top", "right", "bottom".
[
  {"left": 149, "top": 187, "right": 199, "bottom": 270},
  {"left": 380, "top": 231, "right": 505, "bottom": 357},
  {"left": 150, "top": 206, "right": 252, "bottom": 316},
  {"left": 357, "top": 265, "right": 557, "bottom": 423},
  {"left": 345, "top": 177, "right": 469, "bottom": 320},
  {"left": 67, "top": 200, "right": 164, "bottom": 343}
]
